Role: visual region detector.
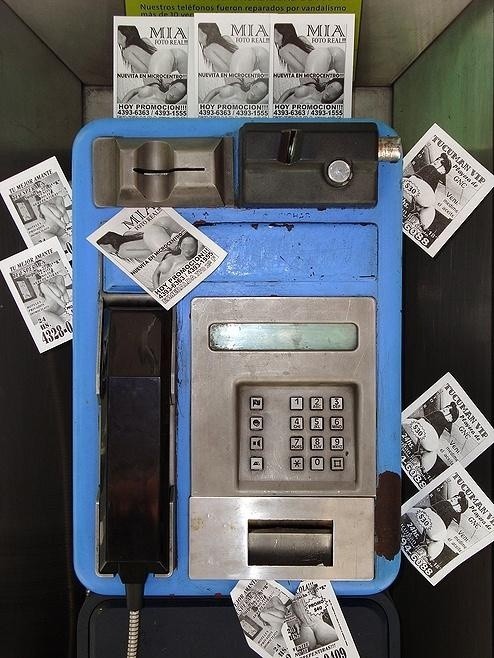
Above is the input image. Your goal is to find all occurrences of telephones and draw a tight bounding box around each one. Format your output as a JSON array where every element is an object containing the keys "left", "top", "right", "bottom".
[{"left": 72, "top": 117, "right": 405, "bottom": 600}]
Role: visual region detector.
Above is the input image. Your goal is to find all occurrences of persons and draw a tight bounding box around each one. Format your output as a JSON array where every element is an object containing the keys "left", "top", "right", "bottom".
[
  {"left": 150, "top": 235, "right": 201, "bottom": 289},
  {"left": 245, "top": 590, "right": 288, "bottom": 652},
  {"left": 31, "top": 181, "right": 73, "bottom": 244},
  {"left": 32, "top": 258, "right": 71, "bottom": 338},
  {"left": 116, "top": 24, "right": 345, "bottom": 104},
  {"left": 398, "top": 150, "right": 452, "bottom": 232},
  {"left": 97, "top": 214, "right": 184, "bottom": 259},
  {"left": 403, "top": 399, "right": 460, "bottom": 478},
  {"left": 291, "top": 584, "right": 340, "bottom": 648},
  {"left": 405, "top": 490, "right": 469, "bottom": 562}
]
